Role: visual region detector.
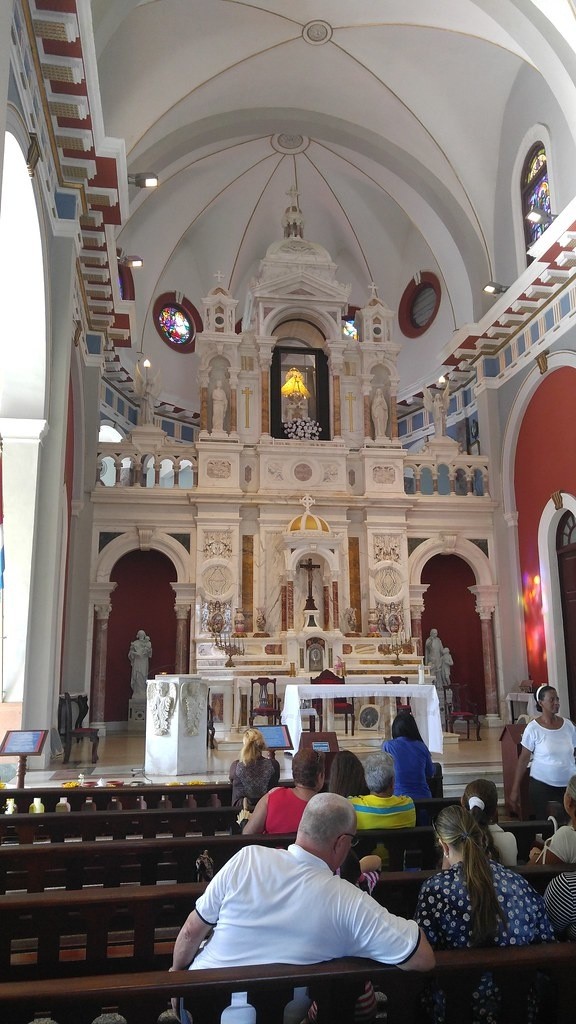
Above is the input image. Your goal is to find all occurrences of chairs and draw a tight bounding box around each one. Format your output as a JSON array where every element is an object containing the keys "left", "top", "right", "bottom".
[
  {"left": 443, "top": 682, "right": 482, "bottom": 741},
  {"left": 63, "top": 692, "right": 100, "bottom": 764},
  {"left": 249, "top": 677, "right": 281, "bottom": 727},
  {"left": 310, "top": 669, "right": 356, "bottom": 736}
]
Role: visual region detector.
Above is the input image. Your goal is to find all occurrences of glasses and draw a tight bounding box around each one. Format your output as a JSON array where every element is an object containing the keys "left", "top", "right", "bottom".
[{"left": 337, "top": 833, "right": 361, "bottom": 848}]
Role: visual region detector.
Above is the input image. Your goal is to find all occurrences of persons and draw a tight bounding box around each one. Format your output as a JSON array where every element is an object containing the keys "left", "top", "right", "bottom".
[
  {"left": 211, "top": 379, "right": 228, "bottom": 433},
  {"left": 307, "top": 851, "right": 382, "bottom": 1024},
  {"left": 128, "top": 630, "right": 152, "bottom": 699},
  {"left": 169, "top": 792, "right": 435, "bottom": 1024},
  {"left": 182, "top": 682, "right": 205, "bottom": 736},
  {"left": 134, "top": 362, "right": 162, "bottom": 425},
  {"left": 543, "top": 871, "right": 576, "bottom": 943},
  {"left": 242, "top": 712, "right": 436, "bottom": 871},
  {"left": 529, "top": 775, "right": 576, "bottom": 866},
  {"left": 372, "top": 388, "right": 390, "bottom": 441},
  {"left": 422, "top": 381, "right": 450, "bottom": 438},
  {"left": 229, "top": 728, "right": 280, "bottom": 835},
  {"left": 442, "top": 779, "right": 517, "bottom": 869},
  {"left": 415, "top": 803, "right": 562, "bottom": 1024},
  {"left": 148, "top": 682, "right": 177, "bottom": 736},
  {"left": 425, "top": 628, "right": 453, "bottom": 690},
  {"left": 509, "top": 685, "right": 576, "bottom": 861}
]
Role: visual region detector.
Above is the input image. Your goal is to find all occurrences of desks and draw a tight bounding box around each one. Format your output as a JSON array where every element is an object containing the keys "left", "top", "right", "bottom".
[
  {"left": 280, "top": 684, "right": 444, "bottom": 757},
  {"left": 505, "top": 693, "right": 535, "bottom": 724}
]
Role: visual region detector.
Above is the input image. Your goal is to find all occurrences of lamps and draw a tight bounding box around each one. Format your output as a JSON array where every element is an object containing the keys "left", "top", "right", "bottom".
[
  {"left": 525, "top": 208, "right": 558, "bottom": 223},
  {"left": 128, "top": 172, "right": 158, "bottom": 189},
  {"left": 152, "top": 292, "right": 203, "bottom": 354},
  {"left": 137, "top": 350, "right": 151, "bottom": 368},
  {"left": 482, "top": 282, "right": 510, "bottom": 294},
  {"left": 117, "top": 254, "right": 144, "bottom": 267}
]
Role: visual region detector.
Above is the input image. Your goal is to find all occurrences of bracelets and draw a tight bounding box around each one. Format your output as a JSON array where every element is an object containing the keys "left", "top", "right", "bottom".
[{"left": 530, "top": 852, "right": 540, "bottom": 859}]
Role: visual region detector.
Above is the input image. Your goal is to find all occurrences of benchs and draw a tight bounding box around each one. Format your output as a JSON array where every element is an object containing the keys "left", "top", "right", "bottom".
[{"left": 0, "top": 783, "right": 576, "bottom": 1024}]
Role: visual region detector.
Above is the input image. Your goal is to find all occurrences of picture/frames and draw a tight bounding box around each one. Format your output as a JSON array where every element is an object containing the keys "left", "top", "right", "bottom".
[{"left": 469, "top": 440, "right": 480, "bottom": 455}]
